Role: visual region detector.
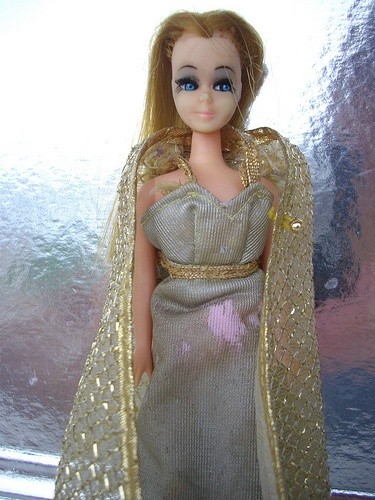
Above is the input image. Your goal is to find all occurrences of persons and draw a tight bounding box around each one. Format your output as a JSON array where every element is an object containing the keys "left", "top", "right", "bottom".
[{"left": 55, "top": 11, "right": 331, "bottom": 500}]
